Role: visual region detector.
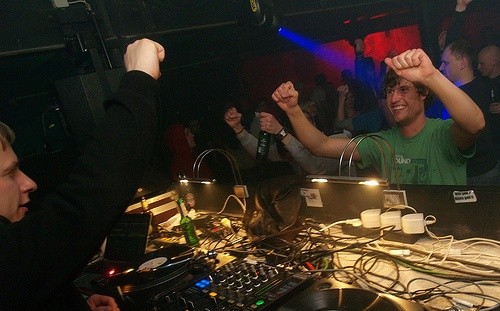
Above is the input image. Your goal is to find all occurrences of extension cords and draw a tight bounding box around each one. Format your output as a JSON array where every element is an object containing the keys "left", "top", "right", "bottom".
[{"left": 343, "top": 222, "right": 421, "bottom": 244}]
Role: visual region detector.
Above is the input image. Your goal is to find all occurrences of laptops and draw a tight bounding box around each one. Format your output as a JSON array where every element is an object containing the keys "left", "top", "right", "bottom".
[{"left": 73, "top": 211, "right": 153, "bottom": 288}]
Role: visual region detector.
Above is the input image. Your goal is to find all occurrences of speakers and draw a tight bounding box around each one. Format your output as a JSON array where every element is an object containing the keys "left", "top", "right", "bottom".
[{"left": 55, "top": 66, "right": 127, "bottom": 149}]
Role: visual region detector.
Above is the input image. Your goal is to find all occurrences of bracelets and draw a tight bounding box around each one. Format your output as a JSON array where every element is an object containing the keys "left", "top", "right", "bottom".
[
  {"left": 275, "top": 128, "right": 290, "bottom": 142},
  {"left": 235, "top": 127, "right": 244, "bottom": 134}
]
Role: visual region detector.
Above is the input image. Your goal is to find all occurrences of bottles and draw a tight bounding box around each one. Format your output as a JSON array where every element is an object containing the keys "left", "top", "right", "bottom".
[
  {"left": 177, "top": 199, "right": 199, "bottom": 247},
  {"left": 488, "top": 88, "right": 498, "bottom": 118},
  {"left": 140, "top": 196, "right": 160, "bottom": 240},
  {"left": 255, "top": 130, "right": 271, "bottom": 159}
]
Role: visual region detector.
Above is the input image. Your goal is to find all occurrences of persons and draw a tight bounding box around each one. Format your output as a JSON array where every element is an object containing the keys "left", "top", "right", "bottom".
[
  {"left": 0, "top": 37, "right": 165, "bottom": 311},
  {"left": 172, "top": 0, "right": 500, "bottom": 186}
]
